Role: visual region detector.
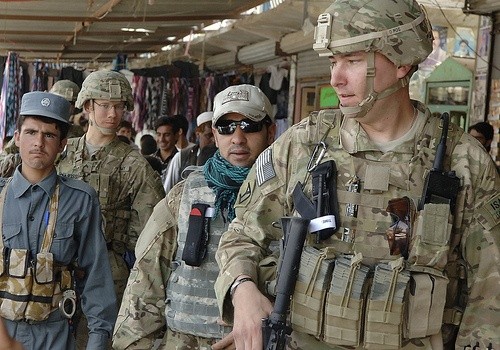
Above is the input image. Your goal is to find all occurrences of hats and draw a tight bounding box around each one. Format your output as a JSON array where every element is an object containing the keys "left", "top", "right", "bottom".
[
  {"left": 197, "top": 111, "right": 213, "bottom": 127},
  {"left": 20, "top": 92, "right": 75, "bottom": 126},
  {"left": 212, "top": 84, "right": 276, "bottom": 128}
]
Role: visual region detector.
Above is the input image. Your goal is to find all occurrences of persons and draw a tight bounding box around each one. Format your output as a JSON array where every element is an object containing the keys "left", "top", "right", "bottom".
[
  {"left": 468, "top": 122, "right": 494, "bottom": 153},
  {"left": 140, "top": 135, "right": 157, "bottom": 155},
  {"left": 0, "top": 79, "right": 88, "bottom": 178},
  {"left": 213, "top": 0, "right": 500, "bottom": 350},
  {"left": 173, "top": 115, "right": 189, "bottom": 151},
  {"left": 116, "top": 121, "right": 131, "bottom": 141},
  {"left": 112, "top": 84, "right": 277, "bottom": 350},
  {"left": 164, "top": 112, "right": 215, "bottom": 193},
  {"left": 0, "top": 90, "right": 118, "bottom": 350},
  {"left": 149, "top": 115, "right": 179, "bottom": 178},
  {"left": 0, "top": 69, "right": 166, "bottom": 350}
]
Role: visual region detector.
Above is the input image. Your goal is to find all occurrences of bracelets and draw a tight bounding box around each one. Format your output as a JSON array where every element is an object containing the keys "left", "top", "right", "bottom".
[{"left": 230, "top": 278, "right": 255, "bottom": 299}]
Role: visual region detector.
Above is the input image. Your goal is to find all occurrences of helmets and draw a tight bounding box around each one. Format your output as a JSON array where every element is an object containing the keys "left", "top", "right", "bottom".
[
  {"left": 49, "top": 80, "right": 81, "bottom": 102},
  {"left": 313, "top": 0, "right": 429, "bottom": 67},
  {"left": 75, "top": 72, "right": 134, "bottom": 112}
]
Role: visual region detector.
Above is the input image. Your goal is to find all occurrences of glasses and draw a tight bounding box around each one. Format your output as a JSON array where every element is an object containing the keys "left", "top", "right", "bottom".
[
  {"left": 216, "top": 117, "right": 271, "bottom": 134},
  {"left": 93, "top": 100, "right": 128, "bottom": 113}
]
